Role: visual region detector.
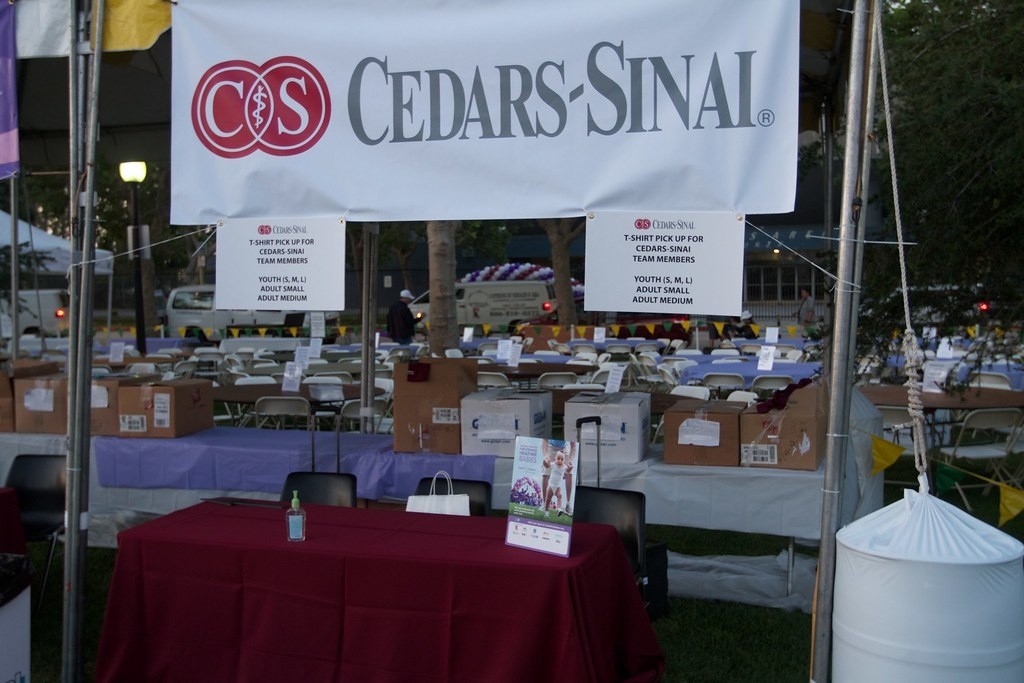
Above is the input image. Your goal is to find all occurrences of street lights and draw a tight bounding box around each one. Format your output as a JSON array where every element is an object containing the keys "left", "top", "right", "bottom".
[{"left": 119, "top": 160, "right": 147, "bottom": 356}]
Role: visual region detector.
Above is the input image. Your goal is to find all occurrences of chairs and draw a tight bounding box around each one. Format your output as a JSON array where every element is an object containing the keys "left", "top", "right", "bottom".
[
  {"left": 17, "top": 338, "right": 101, "bottom": 355},
  {"left": 1, "top": 334, "right": 1024, "bottom": 591}
]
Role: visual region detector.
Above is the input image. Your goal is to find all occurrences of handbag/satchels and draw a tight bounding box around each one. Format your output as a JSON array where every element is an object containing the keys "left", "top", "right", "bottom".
[{"left": 405, "top": 470, "right": 471, "bottom": 516}]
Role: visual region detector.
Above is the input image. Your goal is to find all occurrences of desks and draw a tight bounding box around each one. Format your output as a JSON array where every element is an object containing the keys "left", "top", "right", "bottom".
[
  {"left": 1, "top": 424, "right": 1024, "bottom": 683},
  {"left": 894, "top": 337, "right": 974, "bottom": 351},
  {"left": 567, "top": 339, "right": 665, "bottom": 355},
  {"left": 859, "top": 383, "right": 1024, "bottom": 449},
  {"left": 956, "top": 363, "right": 1024, "bottom": 393},
  {"left": 653, "top": 355, "right": 758, "bottom": 367},
  {"left": 114, "top": 338, "right": 201, "bottom": 354},
  {"left": 238, "top": 362, "right": 388, "bottom": 376},
  {"left": 732, "top": 339, "right": 819, "bottom": 352},
  {"left": 679, "top": 362, "right": 823, "bottom": 392},
  {"left": 517, "top": 353, "right": 573, "bottom": 365},
  {"left": 651, "top": 393, "right": 695, "bottom": 414},
  {"left": 161, "top": 347, "right": 255, "bottom": 366},
  {"left": 327, "top": 344, "right": 418, "bottom": 360},
  {"left": 460, "top": 339, "right": 504, "bottom": 348},
  {"left": 888, "top": 356, "right": 967, "bottom": 370},
  {"left": 218, "top": 337, "right": 310, "bottom": 358},
  {"left": 213, "top": 383, "right": 383, "bottom": 406},
  {"left": 479, "top": 361, "right": 598, "bottom": 386}
]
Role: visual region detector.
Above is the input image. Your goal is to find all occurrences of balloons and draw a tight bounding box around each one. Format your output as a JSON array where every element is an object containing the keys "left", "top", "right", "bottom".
[{"left": 460, "top": 263, "right": 584, "bottom": 295}]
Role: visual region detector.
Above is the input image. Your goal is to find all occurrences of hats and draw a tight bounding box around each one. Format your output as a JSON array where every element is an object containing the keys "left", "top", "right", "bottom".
[{"left": 400, "top": 290, "right": 415, "bottom": 300}]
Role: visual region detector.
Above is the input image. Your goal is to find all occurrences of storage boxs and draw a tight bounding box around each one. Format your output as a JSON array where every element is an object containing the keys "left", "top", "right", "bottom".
[
  {"left": 90, "top": 377, "right": 163, "bottom": 435},
  {"left": 0, "top": 359, "right": 60, "bottom": 433},
  {"left": 740, "top": 380, "right": 831, "bottom": 471},
  {"left": 117, "top": 378, "right": 215, "bottom": 437},
  {"left": 563, "top": 392, "right": 652, "bottom": 463},
  {"left": 393, "top": 358, "right": 478, "bottom": 453},
  {"left": 461, "top": 388, "right": 554, "bottom": 456},
  {"left": 15, "top": 377, "right": 68, "bottom": 435},
  {"left": 664, "top": 399, "right": 748, "bottom": 468}
]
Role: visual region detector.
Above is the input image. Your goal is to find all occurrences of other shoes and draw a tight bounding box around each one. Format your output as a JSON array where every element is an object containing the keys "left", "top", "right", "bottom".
[
  {"left": 538, "top": 501, "right": 546, "bottom": 511},
  {"left": 545, "top": 511, "right": 549, "bottom": 517},
  {"left": 564, "top": 505, "right": 573, "bottom": 516},
  {"left": 557, "top": 509, "right": 563, "bottom": 516}
]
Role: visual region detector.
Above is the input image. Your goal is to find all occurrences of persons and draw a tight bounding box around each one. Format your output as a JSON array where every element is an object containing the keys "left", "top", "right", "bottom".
[
  {"left": 538, "top": 438, "right": 577, "bottom": 518},
  {"left": 797, "top": 288, "right": 818, "bottom": 324},
  {"left": 386, "top": 289, "right": 425, "bottom": 346},
  {"left": 712, "top": 310, "right": 761, "bottom": 339}
]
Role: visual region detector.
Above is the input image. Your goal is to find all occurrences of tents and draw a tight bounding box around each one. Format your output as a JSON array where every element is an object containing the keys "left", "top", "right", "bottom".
[{"left": 0, "top": 210, "right": 114, "bottom": 352}]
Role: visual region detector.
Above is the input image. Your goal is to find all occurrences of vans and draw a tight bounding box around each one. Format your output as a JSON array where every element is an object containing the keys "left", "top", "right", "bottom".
[
  {"left": 877, "top": 281, "right": 989, "bottom": 325},
  {"left": 407, "top": 278, "right": 558, "bottom": 328},
  {"left": 164, "top": 283, "right": 340, "bottom": 345},
  {"left": 0, "top": 288, "right": 73, "bottom": 340}
]
